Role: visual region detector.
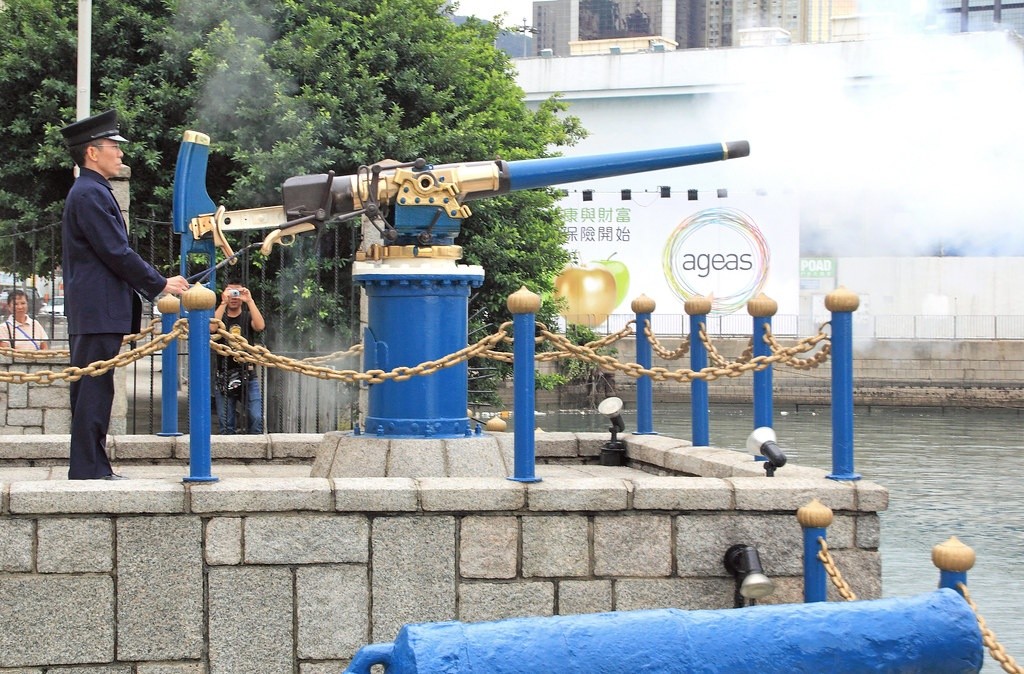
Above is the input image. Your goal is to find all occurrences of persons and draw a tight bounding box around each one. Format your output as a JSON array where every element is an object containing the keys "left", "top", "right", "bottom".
[
  {"left": 209, "top": 279, "right": 265, "bottom": 434},
  {"left": 0, "top": 290, "right": 49, "bottom": 351},
  {"left": 59, "top": 110, "right": 189, "bottom": 480}
]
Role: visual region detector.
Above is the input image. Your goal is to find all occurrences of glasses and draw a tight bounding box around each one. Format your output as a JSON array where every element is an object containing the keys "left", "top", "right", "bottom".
[{"left": 96, "top": 142, "right": 121, "bottom": 148}]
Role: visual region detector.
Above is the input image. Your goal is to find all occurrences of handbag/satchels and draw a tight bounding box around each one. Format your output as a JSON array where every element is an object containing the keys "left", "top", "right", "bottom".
[{"left": 219, "top": 372, "right": 249, "bottom": 399}]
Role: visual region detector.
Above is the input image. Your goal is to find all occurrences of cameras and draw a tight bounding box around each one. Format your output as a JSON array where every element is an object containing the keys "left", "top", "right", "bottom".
[{"left": 228, "top": 290, "right": 240, "bottom": 297}]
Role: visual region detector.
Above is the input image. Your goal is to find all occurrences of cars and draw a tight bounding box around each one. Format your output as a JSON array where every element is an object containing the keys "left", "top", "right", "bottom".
[
  {"left": 0, "top": 288, "right": 46, "bottom": 317},
  {"left": 38, "top": 295, "right": 65, "bottom": 317},
  {"left": 142, "top": 292, "right": 163, "bottom": 318}
]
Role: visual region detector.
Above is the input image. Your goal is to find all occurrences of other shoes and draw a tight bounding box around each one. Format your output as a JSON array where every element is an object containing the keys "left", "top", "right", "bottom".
[{"left": 100, "top": 473, "right": 131, "bottom": 481}]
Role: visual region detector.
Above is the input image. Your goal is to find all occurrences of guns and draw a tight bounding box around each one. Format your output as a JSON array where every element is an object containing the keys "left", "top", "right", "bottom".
[{"left": 172, "top": 128, "right": 751, "bottom": 259}]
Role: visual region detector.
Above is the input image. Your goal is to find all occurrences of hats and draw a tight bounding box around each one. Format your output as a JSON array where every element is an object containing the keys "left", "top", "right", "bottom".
[{"left": 59, "top": 109, "right": 129, "bottom": 147}]
